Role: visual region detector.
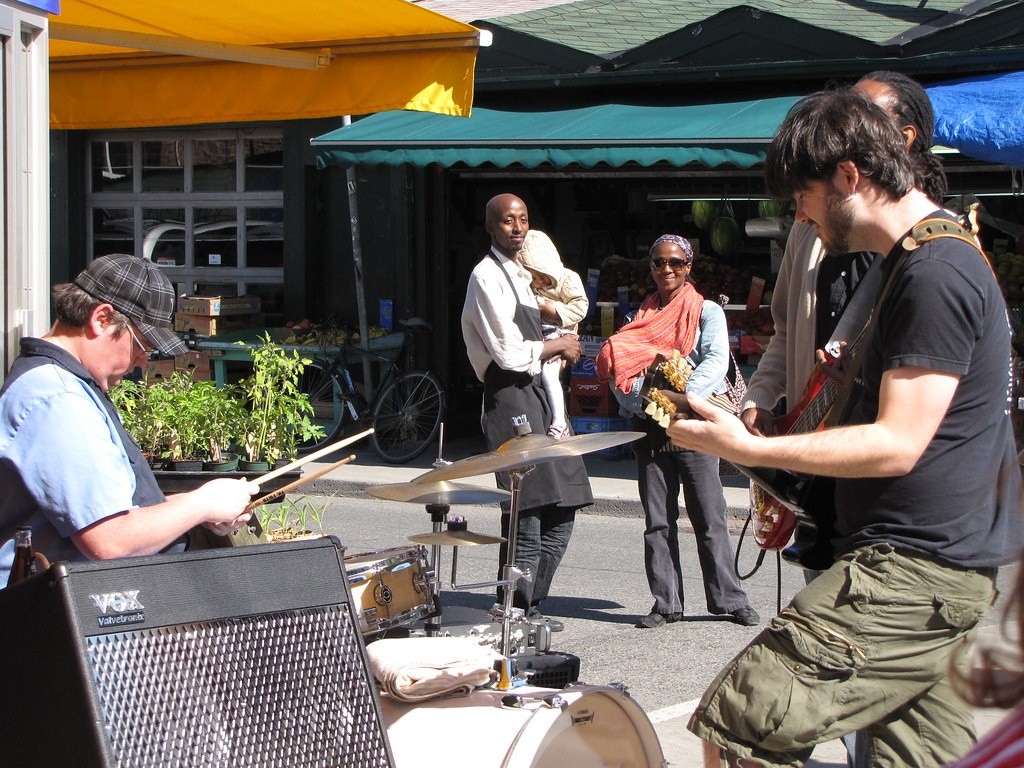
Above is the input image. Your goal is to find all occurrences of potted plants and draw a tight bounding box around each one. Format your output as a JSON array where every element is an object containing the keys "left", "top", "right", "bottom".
[
  {"left": 198, "top": 383, "right": 238, "bottom": 472},
  {"left": 235, "top": 331, "right": 270, "bottom": 472},
  {"left": 272, "top": 341, "right": 328, "bottom": 470},
  {"left": 164, "top": 381, "right": 204, "bottom": 472}
]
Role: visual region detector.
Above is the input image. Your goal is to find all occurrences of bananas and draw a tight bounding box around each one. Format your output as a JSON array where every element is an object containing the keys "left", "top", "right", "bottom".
[{"left": 984, "top": 251, "right": 1024, "bottom": 281}]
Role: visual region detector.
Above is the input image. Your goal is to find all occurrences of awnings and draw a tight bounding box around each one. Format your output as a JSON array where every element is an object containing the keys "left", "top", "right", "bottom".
[
  {"left": 46, "top": 0, "right": 500, "bottom": 126},
  {"left": 307, "top": 84, "right": 831, "bottom": 406}
]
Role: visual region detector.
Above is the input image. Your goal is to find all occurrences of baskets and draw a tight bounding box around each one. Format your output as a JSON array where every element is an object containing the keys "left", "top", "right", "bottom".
[{"left": 522, "top": 665, "right": 571, "bottom": 689}]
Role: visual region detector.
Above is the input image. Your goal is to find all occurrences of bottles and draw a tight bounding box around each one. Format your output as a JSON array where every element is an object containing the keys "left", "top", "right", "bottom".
[
  {"left": 601, "top": 305, "right": 614, "bottom": 337},
  {"left": 7, "top": 526, "right": 39, "bottom": 586}
]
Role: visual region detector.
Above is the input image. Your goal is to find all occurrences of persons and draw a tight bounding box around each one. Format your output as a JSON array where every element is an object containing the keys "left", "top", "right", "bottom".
[
  {"left": 516, "top": 224, "right": 589, "bottom": 440},
  {"left": 0, "top": 252, "right": 261, "bottom": 768},
  {"left": 459, "top": 191, "right": 582, "bottom": 632},
  {"left": 593, "top": 230, "right": 762, "bottom": 626},
  {"left": 663, "top": 87, "right": 1024, "bottom": 766},
  {"left": 739, "top": 69, "right": 946, "bottom": 767}
]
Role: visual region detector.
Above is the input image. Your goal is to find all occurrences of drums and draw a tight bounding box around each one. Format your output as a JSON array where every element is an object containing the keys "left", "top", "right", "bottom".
[
  {"left": 340, "top": 543, "right": 438, "bottom": 637},
  {"left": 377, "top": 681, "right": 667, "bottom": 768}
]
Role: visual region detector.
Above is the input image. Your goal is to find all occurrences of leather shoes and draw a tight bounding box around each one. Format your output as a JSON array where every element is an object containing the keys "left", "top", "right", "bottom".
[{"left": 527, "top": 616, "right": 564, "bottom": 632}]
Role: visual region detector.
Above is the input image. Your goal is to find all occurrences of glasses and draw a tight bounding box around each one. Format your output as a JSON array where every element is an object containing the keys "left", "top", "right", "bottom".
[
  {"left": 98, "top": 302, "right": 155, "bottom": 358},
  {"left": 650, "top": 258, "right": 689, "bottom": 271}
]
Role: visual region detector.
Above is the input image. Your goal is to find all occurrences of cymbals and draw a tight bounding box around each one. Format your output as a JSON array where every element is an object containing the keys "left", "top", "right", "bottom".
[
  {"left": 408, "top": 431, "right": 647, "bottom": 485},
  {"left": 366, "top": 480, "right": 513, "bottom": 505},
  {"left": 407, "top": 529, "right": 508, "bottom": 547}
]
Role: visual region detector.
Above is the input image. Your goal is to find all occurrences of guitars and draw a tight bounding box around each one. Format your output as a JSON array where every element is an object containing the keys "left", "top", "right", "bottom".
[
  {"left": 638, "top": 348, "right": 836, "bottom": 569},
  {"left": 747, "top": 192, "right": 982, "bottom": 553}
]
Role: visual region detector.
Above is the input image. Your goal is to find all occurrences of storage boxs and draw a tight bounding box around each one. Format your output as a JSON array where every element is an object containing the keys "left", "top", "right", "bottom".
[
  {"left": 183, "top": 370, "right": 212, "bottom": 388},
  {"left": 570, "top": 355, "right": 605, "bottom": 376},
  {"left": 379, "top": 296, "right": 393, "bottom": 334},
  {"left": 173, "top": 313, "right": 263, "bottom": 336},
  {"left": 569, "top": 415, "right": 636, "bottom": 460},
  {"left": 142, "top": 360, "right": 174, "bottom": 379},
  {"left": 177, "top": 295, "right": 262, "bottom": 317},
  {"left": 174, "top": 350, "right": 208, "bottom": 369},
  {"left": 571, "top": 374, "right": 620, "bottom": 417}
]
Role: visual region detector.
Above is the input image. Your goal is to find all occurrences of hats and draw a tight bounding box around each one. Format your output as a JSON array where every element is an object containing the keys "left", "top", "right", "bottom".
[{"left": 75, "top": 254, "right": 190, "bottom": 355}]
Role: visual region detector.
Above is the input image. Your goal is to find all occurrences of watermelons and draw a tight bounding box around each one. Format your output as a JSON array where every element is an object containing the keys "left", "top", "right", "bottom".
[
  {"left": 761, "top": 290, "right": 773, "bottom": 304},
  {"left": 757, "top": 200, "right": 782, "bottom": 218},
  {"left": 691, "top": 200, "right": 740, "bottom": 257}
]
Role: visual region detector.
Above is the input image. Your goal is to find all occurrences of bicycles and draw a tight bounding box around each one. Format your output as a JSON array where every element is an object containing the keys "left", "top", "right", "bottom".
[{"left": 270, "top": 313, "right": 448, "bottom": 465}]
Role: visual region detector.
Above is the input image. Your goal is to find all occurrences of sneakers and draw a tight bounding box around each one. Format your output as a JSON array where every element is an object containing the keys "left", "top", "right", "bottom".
[
  {"left": 636, "top": 610, "right": 683, "bottom": 627},
  {"left": 726, "top": 604, "right": 760, "bottom": 625},
  {"left": 547, "top": 425, "right": 570, "bottom": 441}
]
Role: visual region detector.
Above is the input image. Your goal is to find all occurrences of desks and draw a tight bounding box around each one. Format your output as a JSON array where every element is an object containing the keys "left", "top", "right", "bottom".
[{"left": 199, "top": 330, "right": 406, "bottom": 438}]
